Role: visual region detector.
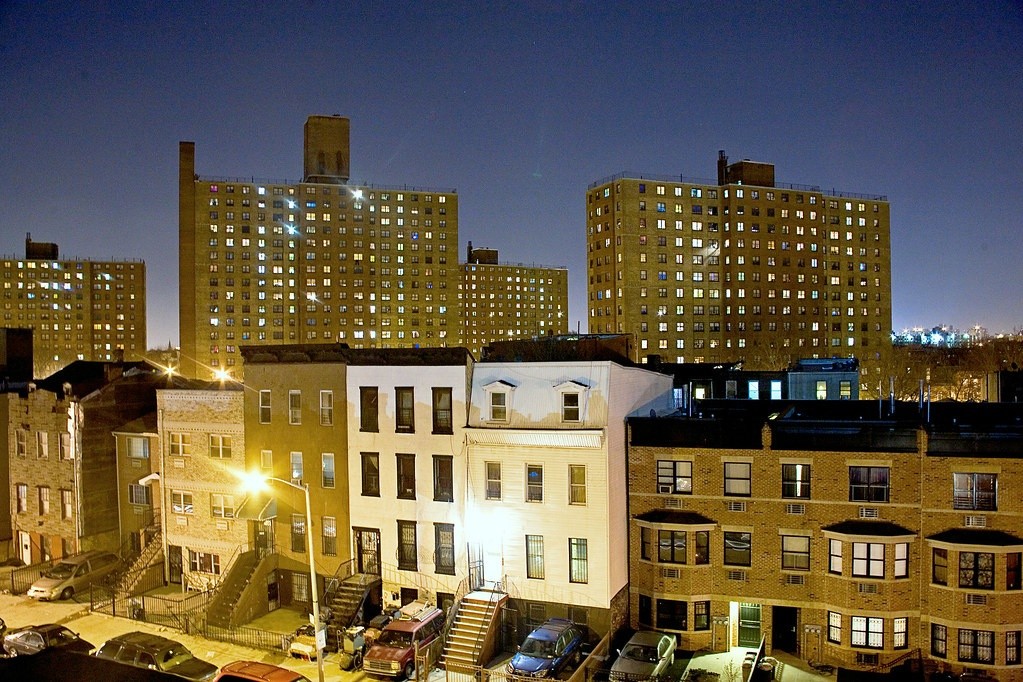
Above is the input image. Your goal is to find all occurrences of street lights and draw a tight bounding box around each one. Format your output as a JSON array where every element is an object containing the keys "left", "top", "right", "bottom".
[{"left": 240, "top": 471, "right": 325, "bottom": 682}]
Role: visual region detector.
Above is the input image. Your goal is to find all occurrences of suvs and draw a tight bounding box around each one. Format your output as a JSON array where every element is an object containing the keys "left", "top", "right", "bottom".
[
  {"left": 88, "top": 631, "right": 220, "bottom": 682},
  {"left": 27, "top": 549, "right": 122, "bottom": 602},
  {"left": 505, "top": 617, "right": 586, "bottom": 682},
  {"left": 212, "top": 660, "right": 313, "bottom": 682},
  {"left": 361, "top": 600, "right": 449, "bottom": 679}
]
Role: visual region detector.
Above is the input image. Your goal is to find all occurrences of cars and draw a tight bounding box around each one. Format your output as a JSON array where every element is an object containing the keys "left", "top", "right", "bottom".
[
  {"left": 3, "top": 622, "right": 97, "bottom": 659},
  {"left": 608, "top": 630, "right": 679, "bottom": 682}
]
